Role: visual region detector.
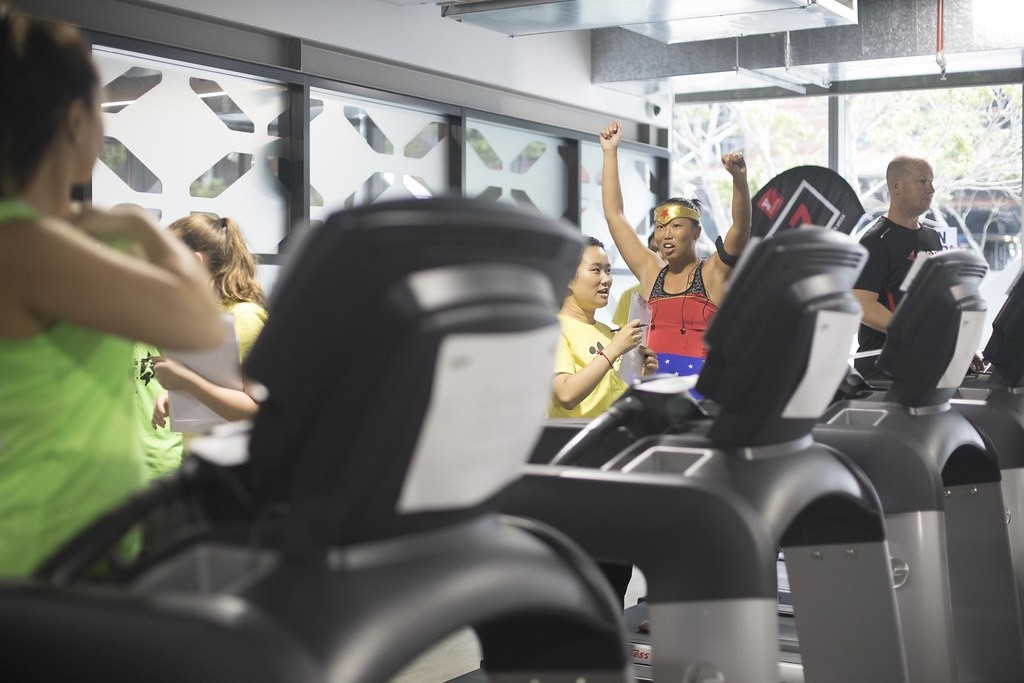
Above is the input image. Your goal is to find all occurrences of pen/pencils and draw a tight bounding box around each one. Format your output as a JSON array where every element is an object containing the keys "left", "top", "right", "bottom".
[{"left": 610, "top": 324, "right": 647, "bottom": 333}]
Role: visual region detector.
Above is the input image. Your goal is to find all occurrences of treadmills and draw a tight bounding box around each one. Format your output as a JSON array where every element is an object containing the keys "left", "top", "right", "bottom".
[
  {"left": 634, "top": 249, "right": 1024, "bottom": 624},
  {"left": 465, "top": 225, "right": 910, "bottom": 683},
  {"left": 612, "top": 248, "right": 1023, "bottom": 683},
  {"left": 0, "top": 198, "right": 635, "bottom": 683}
]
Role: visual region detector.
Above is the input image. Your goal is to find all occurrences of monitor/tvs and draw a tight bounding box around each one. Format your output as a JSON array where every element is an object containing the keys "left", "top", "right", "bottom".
[
  {"left": 697, "top": 226, "right": 870, "bottom": 445},
  {"left": 243, "top": 200, "right": 586, "bottom": 540},
  {"left": 984, "top": 265, "right": 1024, "bottom": 370},
  {"left": 874, "top": 246, "right": 989, "bottom": 404}
]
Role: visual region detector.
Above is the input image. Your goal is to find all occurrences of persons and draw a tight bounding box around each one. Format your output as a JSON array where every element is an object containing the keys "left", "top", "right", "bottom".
[
  {"left": 848, "top": 155, "right": 985, "bottom": 386},
  {"left": 549, "top": 235, "right": 660, "bottom": 431},
  {"left": 610, "top": 229, "right": 671, "bottom": 332},
  {"left": 0, "top": 7, "right": 225, "bottom": 584},
  {"left": 148, "top": 210, "right": 273, "bottom": 469},
  {"left": 600, "top": 121, "right": 752, "bottom": 415}
]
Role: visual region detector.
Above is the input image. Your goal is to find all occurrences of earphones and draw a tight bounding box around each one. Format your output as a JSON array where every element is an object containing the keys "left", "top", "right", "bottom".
[
  {"left": 651, "top": 323, "right": 655, "bottom": 330},
  {"left": 680, "top": 328, "right": 685, "bottom": 334}
]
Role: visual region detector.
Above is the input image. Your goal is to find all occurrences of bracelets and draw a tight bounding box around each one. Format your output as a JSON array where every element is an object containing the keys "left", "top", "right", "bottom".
[{"left": 597, "top": 350, "right": 615, "bottom": 369}]
